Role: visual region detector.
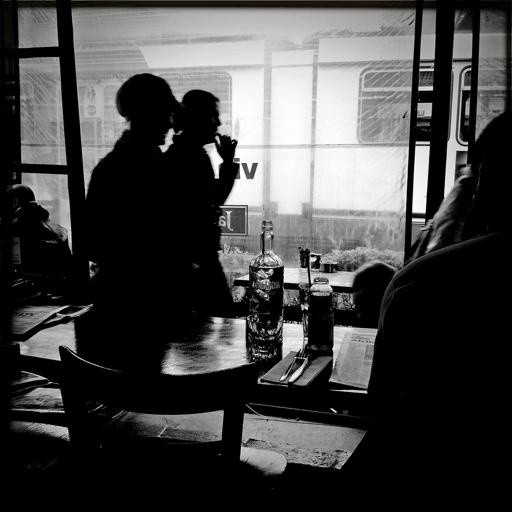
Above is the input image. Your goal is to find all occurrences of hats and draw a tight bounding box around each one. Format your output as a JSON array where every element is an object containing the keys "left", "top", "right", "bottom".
[{"left": 116, "top": 73, "right": 182, "bottom": 115}]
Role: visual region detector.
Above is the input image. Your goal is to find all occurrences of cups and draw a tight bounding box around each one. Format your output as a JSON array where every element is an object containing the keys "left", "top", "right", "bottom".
[
  {"left": 297, "top": 282, "right": 311, "bottom": 344},
  {"left": 245, "top": 313, "right": 284, "bottom": 365}
]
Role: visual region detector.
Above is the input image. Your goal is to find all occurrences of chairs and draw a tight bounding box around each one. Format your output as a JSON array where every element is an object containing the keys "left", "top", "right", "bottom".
[
  {"left": 58, "top": 343, "right": 287, "bottom": 467},
  {"left": 344, "top": 259, "right": 400, "bottom": 329}
]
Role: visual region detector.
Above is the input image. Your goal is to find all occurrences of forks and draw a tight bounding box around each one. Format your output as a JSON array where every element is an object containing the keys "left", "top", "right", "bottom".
[{"left": 277, "top": 348, "right": 308, "bottom": 383}]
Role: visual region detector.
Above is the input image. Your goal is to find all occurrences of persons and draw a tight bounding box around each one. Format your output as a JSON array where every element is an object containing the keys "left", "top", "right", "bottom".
[
  {"left": 415, "top": 139, "right": 488, "bottom": 256},
  {"left": 82, "top": 74, "right": 188, "bottom": 311},
  {"left": 8, "top": 183, "right": 81, "bottom": 302},
  {"left": 366, "top": 111, "right": 512, "bottom": 512},
  {"left": 166, "top": 89, "right": 242, "bottom": 318}
]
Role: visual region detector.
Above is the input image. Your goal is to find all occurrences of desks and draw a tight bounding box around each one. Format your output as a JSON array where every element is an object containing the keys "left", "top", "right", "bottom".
[
  {"left": 7, "top": 305, "right": 380, "bottom": 429},
  {"left": 232, "top": 266, "right": 357, "bottom": 293}
]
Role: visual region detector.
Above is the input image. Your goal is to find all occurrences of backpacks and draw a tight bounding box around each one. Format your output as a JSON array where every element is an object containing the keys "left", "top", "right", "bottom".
[{"left": 404, "top": 221, "right": 432, "bottom": 264}]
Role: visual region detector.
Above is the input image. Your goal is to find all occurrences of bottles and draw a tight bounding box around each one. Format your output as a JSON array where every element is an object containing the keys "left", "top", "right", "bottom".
[
  {"left": 246, "top": 221, "right": 283, "bottom": 360},
  {"left": 306, "top": 278, "right": 334, "bottom": 353}
]
